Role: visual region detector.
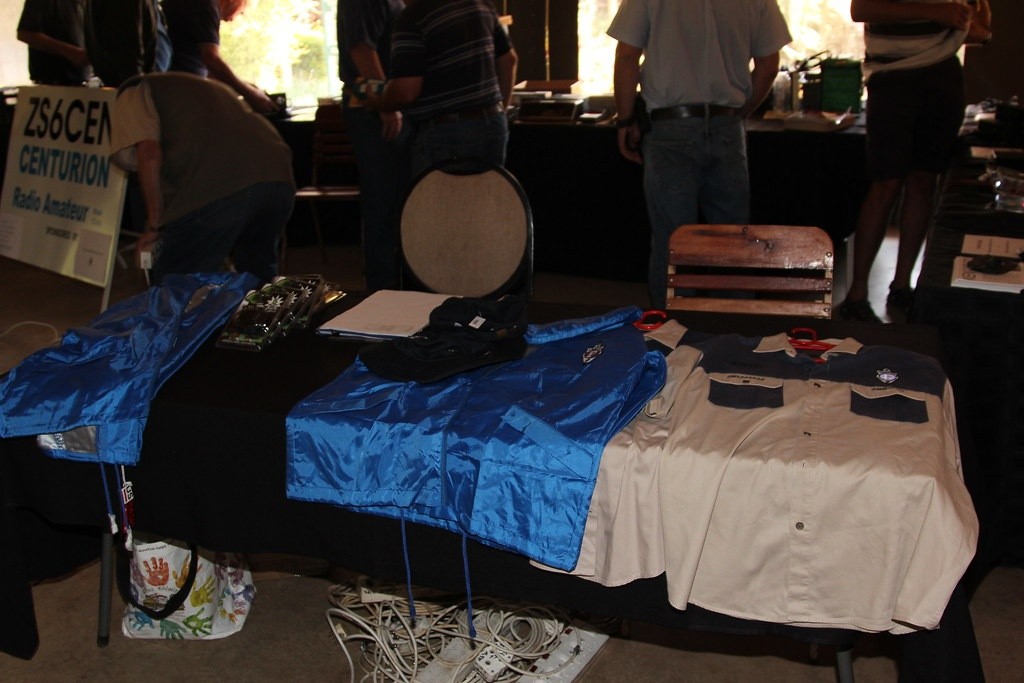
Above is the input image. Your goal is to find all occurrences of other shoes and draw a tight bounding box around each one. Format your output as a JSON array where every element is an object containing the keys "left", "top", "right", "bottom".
[
  {"left": 889, "top": 283, "right": 916, "bottom": 311},
  {"left": 834, "top": 297, "right": 879, "bottom": 326}
]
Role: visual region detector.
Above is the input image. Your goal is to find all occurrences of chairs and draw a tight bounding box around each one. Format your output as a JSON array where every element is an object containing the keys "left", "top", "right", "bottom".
[
  {"left": 384, "top": 153, "right": 544, "bottom": 327},
  {"left": 281, "top": 100, "right": 376, "bottom": 267},
  {"left": 663, "top": 219, "right": 837, "bottom": 340}
]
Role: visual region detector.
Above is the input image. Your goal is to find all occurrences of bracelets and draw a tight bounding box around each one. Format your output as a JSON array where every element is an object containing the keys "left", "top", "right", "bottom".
[{"left": 617, "top": 114, "right": 635, "bottom": 126}]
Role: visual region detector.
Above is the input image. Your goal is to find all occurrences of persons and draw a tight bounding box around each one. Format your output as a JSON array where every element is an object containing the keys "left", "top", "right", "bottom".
[
  {"left": 18, "top": 0, "right": 281, "bottom": 243},
  {"left": 337, "top": 0, "right": 518, "bottom": 288},
  {"left": 838, "top": 0, "right": 993, "bottom": 320},
  {"left": 116, "top": 69, "right": 296, "bottom": 286},
  {"left": 609, "top": 0, "right": 791, "bottom": 308}
]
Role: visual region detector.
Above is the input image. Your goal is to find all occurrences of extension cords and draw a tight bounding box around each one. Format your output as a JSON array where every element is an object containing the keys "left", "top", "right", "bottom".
[
  {"left": 516, "top": 619, "right": 610, "bottom": 683},
  {"left": 414, "top": 607, "right": 517, "bottom": 683}
]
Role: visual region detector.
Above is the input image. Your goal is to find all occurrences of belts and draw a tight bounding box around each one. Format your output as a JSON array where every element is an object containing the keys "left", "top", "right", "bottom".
[
  {"left": 649, "top": 103, "right": 738, "bottom": 119},
  {"left": 417, "top": 100, "right": 507, "bottom": 127}
]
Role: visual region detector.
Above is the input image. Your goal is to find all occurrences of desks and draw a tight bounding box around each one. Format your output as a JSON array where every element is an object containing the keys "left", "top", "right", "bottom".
[{"left": 1, "top": 98, "right": 1024, "bottom": 682}]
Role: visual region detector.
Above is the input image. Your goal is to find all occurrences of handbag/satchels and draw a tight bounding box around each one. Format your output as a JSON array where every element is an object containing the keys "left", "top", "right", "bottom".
[{"left": 119, "top": 534, "right": 255, "bottom": 639}]
